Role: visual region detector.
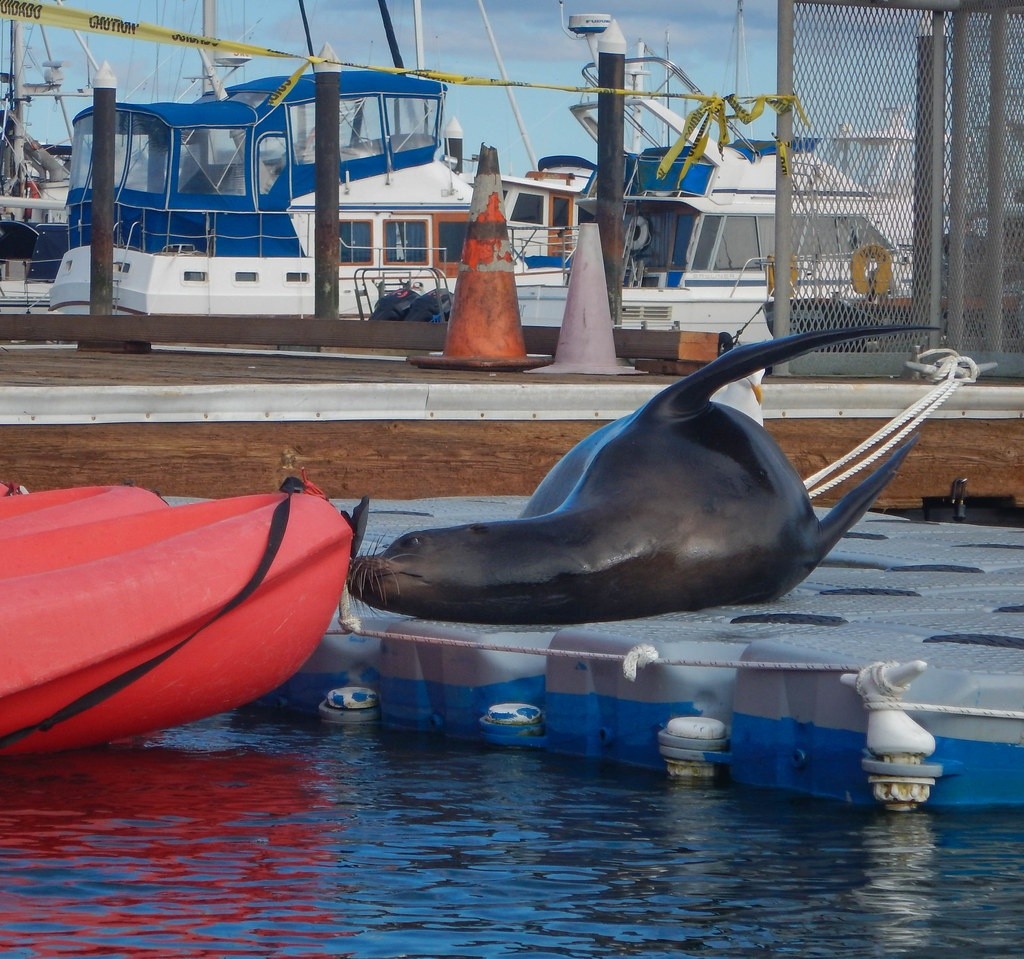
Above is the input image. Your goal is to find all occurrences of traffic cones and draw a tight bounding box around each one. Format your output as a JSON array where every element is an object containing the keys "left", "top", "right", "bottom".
[
  {"left": 523, "top": 223, "right": 649, "bottom": 375},
  {"left": 405, "top": 142, "right": 552, "bottom": 371}
]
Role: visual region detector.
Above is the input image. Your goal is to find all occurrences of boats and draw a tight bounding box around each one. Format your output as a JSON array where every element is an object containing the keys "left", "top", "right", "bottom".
[
  {"left": 0, "top": 478, "right": 352, "bottom": 758},
  {"left": 49, "top": 70, "right": 595, "bottom": 319},
  {"left": 353, "top": 12, "right": 914, "bottom": 348}
]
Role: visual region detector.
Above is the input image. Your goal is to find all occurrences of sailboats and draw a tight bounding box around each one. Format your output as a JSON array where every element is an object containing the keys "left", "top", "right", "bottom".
[{"left": 0, "top": 0, "right": 101, "bottom": 313}]
